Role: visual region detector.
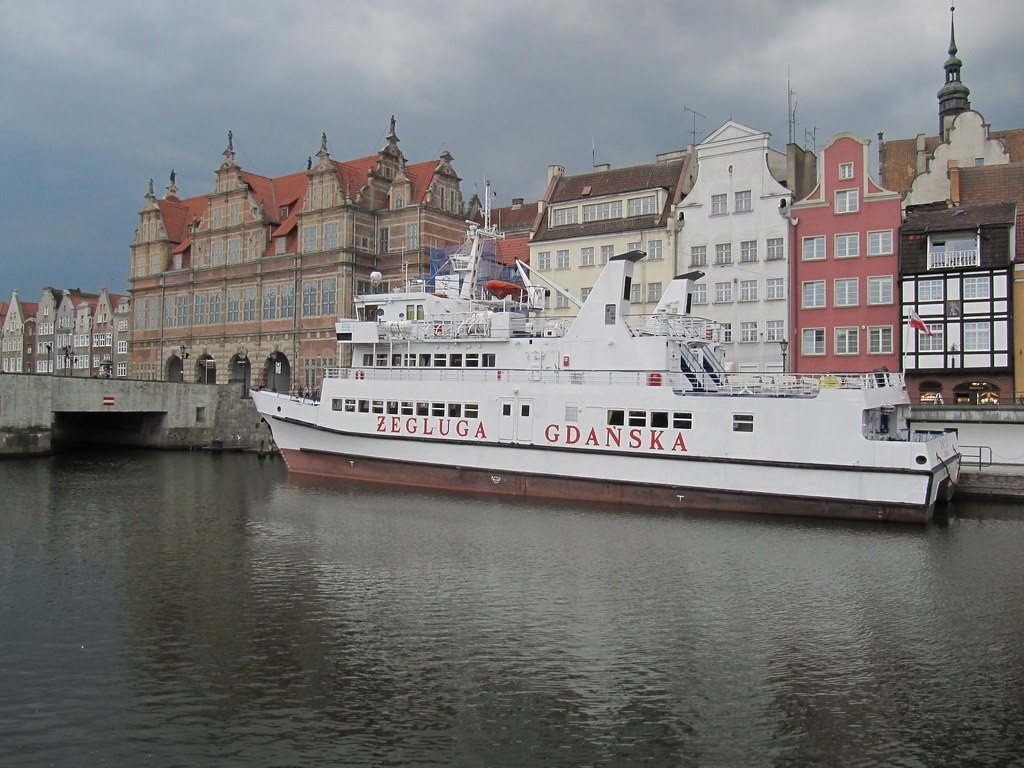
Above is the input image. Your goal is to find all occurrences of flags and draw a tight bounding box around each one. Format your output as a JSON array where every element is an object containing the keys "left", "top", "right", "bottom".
[{"left": 910, "top": 309, "right": 933, "bottom": 337}]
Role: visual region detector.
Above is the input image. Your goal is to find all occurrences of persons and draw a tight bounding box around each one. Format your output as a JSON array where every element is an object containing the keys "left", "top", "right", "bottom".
[
  {"left": 320, "top": 132, "right": 328, "bottom": 150},
  {"left": 307, "top": 157, "right": 312, "bottom": 170},
  {"left": 149, "top": 179, "right": 154, "bottom": 194},
  {"left": 93, "top": 372, "right": 108, "bottom": 379},
  {"left": 228, "top": 131, "right": 233, "bottom": 148},
  {"left": 297, "top": 384, "right": 316, "bottom": 398},
  {"left": 170, "top": 170, "right": 176, "bottom": 182},
  {"left": 390, "top": 116, "right": 396, "bottom": 131}
]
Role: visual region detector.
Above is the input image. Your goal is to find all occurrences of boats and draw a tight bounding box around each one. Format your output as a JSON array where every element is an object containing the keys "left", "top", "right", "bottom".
[{"left": 249, "top": 181, "right": 961, "bottom": 524}]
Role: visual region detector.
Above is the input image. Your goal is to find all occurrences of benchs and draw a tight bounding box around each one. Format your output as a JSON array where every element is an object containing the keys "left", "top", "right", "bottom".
[{"left": 727, "top": 375, "right": 862, "bottom": 396}]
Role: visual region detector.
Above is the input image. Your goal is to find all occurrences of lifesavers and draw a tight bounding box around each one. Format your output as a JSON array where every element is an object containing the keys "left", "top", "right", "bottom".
[{"left": 355, "top": 371, "right": 364, "bottom": 380}]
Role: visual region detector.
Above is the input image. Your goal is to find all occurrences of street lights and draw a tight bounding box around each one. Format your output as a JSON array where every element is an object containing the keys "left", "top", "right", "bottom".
[
  {"left": 68, "top": 350, "right": 77, "bottom": 376},
  {"left": 236, "top": 348, "right": 248, "bottom": 399},
  {"left": 44, "top": 343, "right": 52, "bottom": 376},
  {"left": 268, "top": 351, "right": 276, "bottom": 392},
  {"left": 181, "top": 345, "right": 189, "bottom": 382},
  {"left": 63, "top": 345, "right": 71, "bottom": 376}
]
